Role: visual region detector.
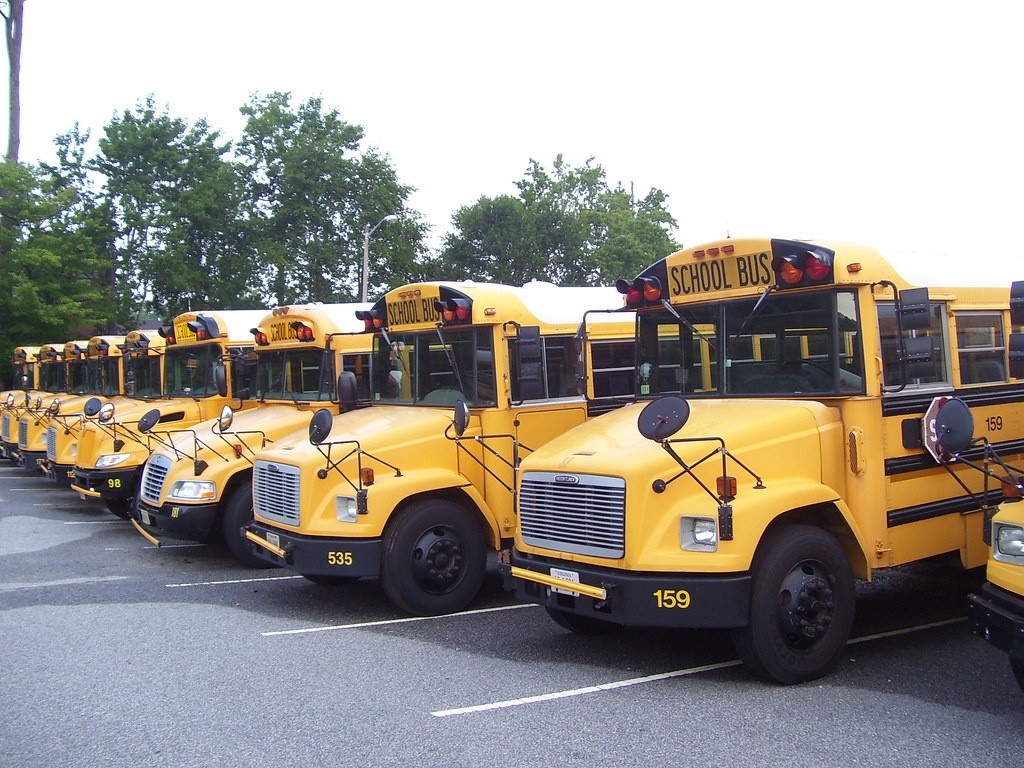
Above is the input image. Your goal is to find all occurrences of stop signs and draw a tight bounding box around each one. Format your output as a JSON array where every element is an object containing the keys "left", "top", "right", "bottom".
[{"left": 924, "top": 396, "right": 961, "bottom": 463}]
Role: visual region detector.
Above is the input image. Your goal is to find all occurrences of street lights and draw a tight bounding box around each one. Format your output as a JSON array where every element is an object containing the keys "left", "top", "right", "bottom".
[{"left": 362, "top": 215, "right": 399, "bottom": 303}]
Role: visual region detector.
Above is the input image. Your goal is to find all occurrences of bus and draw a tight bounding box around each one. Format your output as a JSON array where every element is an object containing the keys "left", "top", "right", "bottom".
[
  {"left": 1, "top": 341, "right": 89, "bottom": 489},
  {"left": 238, "top": 272, "right": 850, "bottom": 617},
  {"left": 935, "top": 280, "right": 1024, "bottom": 689},
  {"left": 65, "top": 329, "right": 206, "bottom": 523},
  {"left": 127, "top": 299, "right": 454, "bottom": 572},
  {"left": 444, "top": 233, "right": 1024, "bottom": 686}
]
